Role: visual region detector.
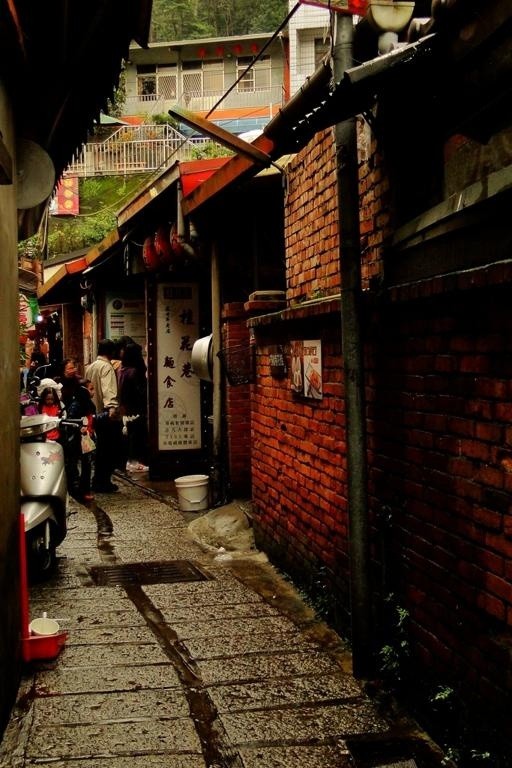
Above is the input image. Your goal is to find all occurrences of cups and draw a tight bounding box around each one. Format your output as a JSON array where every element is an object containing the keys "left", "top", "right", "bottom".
[{"left": 29, "top": 617, "right": 59, "bottom": 636}]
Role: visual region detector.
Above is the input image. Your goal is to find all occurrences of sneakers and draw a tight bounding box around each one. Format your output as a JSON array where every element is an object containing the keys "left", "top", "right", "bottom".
[{"left": 70, "top": 482, "right": 119, "bottom": 497}]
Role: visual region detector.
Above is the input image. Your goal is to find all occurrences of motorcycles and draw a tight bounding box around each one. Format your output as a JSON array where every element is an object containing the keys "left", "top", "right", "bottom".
[{"left": 18, "top": 413, "right": 81, "bottom": 580}]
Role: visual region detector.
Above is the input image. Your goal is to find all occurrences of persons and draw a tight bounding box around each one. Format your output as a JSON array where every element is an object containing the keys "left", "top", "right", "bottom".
[{"left": 20, "top": 334, "right": 148, "bottom": 552}]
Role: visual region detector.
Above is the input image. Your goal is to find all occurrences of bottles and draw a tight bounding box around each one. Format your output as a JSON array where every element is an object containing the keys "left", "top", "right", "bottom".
[{"left": 28, "top": 291, "right": 38, "bottom": 325}]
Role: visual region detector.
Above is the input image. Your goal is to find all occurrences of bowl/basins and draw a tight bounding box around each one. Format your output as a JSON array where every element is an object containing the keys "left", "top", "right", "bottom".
[{"left": 191, "top": 333, "right": 213, "bottom": 382}]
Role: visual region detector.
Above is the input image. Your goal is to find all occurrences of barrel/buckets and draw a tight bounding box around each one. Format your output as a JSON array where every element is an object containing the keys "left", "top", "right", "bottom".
[{"left": 174, "top": 474, "right": 209, "bottom": 512}]
